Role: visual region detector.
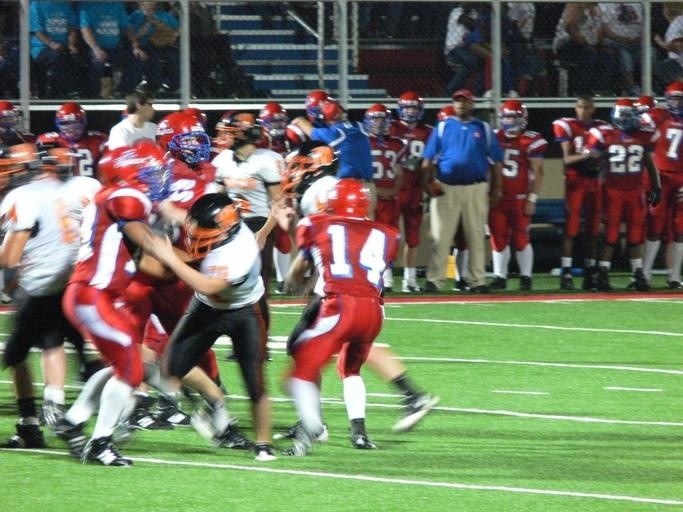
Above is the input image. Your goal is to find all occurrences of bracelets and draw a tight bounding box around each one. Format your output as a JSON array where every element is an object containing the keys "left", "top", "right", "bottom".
[{"left": 525, "top": 192, "right": 538, "bottom": 202}]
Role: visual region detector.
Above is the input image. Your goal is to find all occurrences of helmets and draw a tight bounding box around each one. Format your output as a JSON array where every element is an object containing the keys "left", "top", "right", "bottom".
[
  {"left": 610, "top": 81, "right": 683, "bottom": 135},
  {"left": 498, "top": 100, "right": 529, "bottom": 138},
  {"left": 325, "top": 178, "right": 371, "bottom": 219},
  {"left": 182, "top": 193, "right": 242, "bottom": 261}
]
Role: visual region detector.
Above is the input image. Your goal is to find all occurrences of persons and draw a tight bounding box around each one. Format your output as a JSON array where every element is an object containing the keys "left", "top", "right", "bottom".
[
  {"left": 27, "top": 4, "right": 85, "bottom": 100},
  {"left": 168, "top": 3, "right": 240, "bottom": 99},
  {"left": 552, "top": 82, "right": 682, "bottom": 292},
  {"left": 553, "top": 4, "right": 644, "bottom": 96},
  {"left": 2, "top": 92, "right": 439, "bottom": 466},
  {"left": 445, "top": 4, "right": 547, "bottom": 99},
  {"left": 294, "top": 5, "right": 450, "bottom": 40},
  {"left": 127, "top": 4, "right": 179, "bottom": 98},
  {"left": 75, "top": 4, "right": 147, "bottom": 99},
  {"left": 651, "top": 4, "right": 681, "bottom": 96},
  {"left": 420, "top": 92, "right": 548, "bottom": 290}
]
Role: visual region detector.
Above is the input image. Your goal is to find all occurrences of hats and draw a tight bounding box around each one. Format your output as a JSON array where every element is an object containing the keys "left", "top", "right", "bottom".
[{"left": 451, "top": 89, "right": 474, "bottom": 100}]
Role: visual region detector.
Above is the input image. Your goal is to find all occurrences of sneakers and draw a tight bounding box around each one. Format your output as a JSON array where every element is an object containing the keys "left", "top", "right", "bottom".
[
  {"left": 0, "top": 422, "right": 47, "bottom": 449},
  {"left": 348, "top": 434, "right": 379, "bottom": 450},
  {"left": 393, "top": 392, "right": 440, "bottom": 431},
  {"left": 79, "top": 433, "right": 133, "bottom": 467},
  {"left": 42, "top": 346, "right": 330, "bottom": 457},
  {"left": 52, "top": 417, "right": 89, "bottom": 460}
]
